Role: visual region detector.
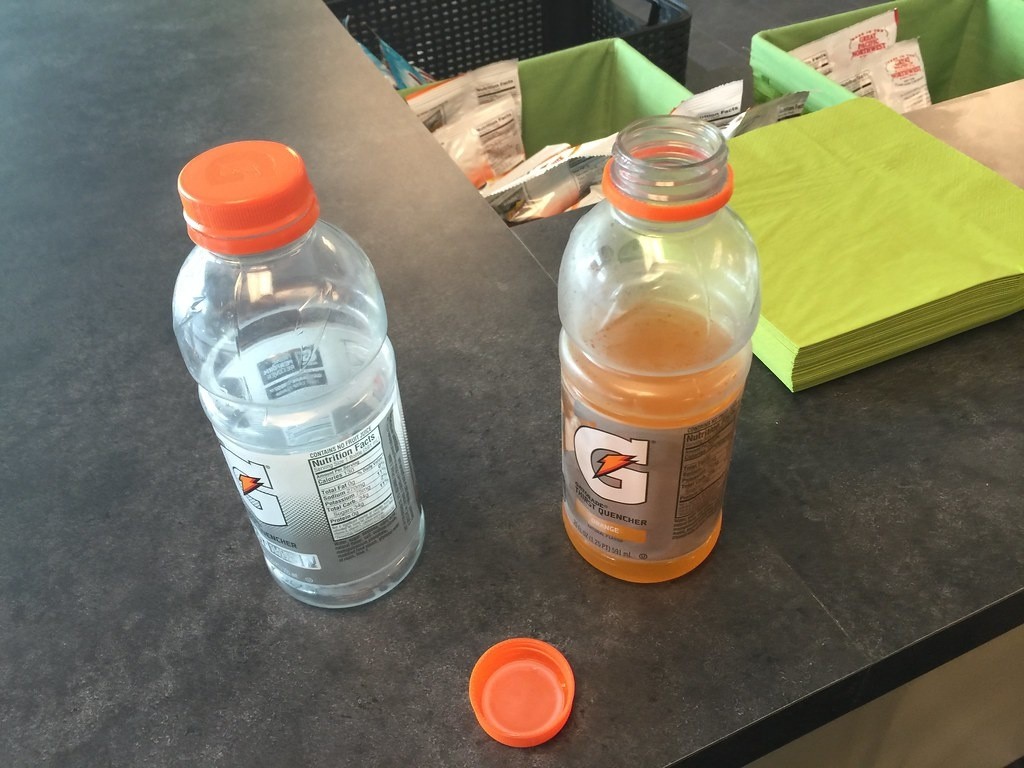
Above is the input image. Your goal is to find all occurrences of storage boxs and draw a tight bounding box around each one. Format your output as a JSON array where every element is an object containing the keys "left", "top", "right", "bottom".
[
  {"left": 397, "top": 38, "right": 694, "bottom": 157},
  {"left": 749, "top": 0, "right": 1024, "bottom": 116},
  {"left": 322, "top": 0, "right": 691, "bottom": 88}
]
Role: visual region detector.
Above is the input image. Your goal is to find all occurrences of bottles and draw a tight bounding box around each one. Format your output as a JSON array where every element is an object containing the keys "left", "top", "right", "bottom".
[
  {"left": 171, "top": 140, "right": 424, "bottom": 609},
  {"left": 554, "top": 116, "right": 762, "bottom": 582}
]
